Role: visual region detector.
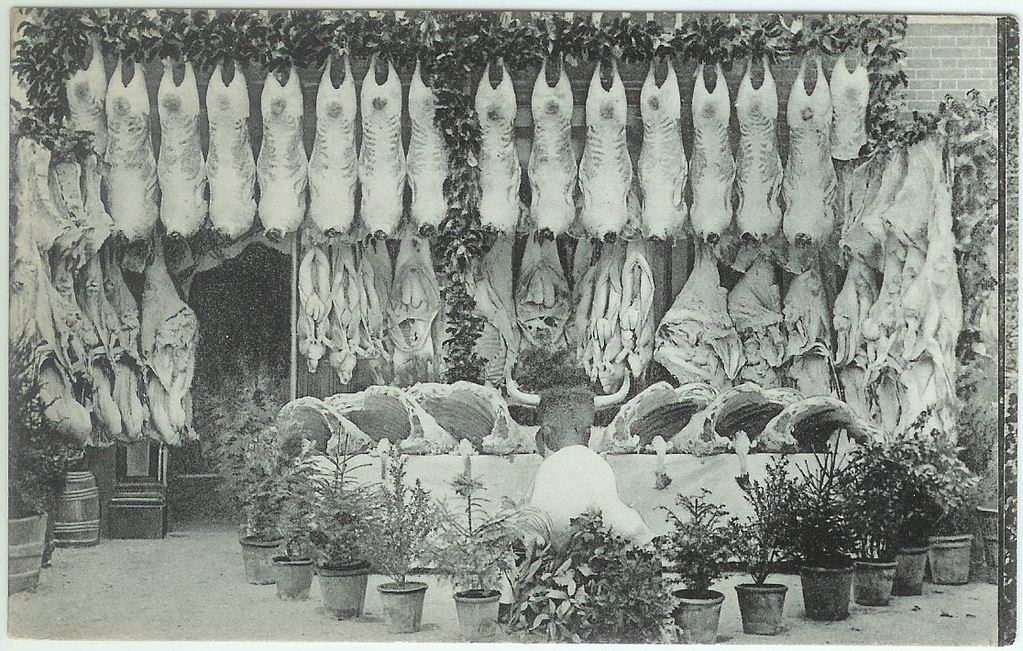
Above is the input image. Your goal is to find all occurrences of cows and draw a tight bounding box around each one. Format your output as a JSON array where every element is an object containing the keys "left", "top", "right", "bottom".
[{"left": 4, "top": 33, "right": 964, "bottom": 454}]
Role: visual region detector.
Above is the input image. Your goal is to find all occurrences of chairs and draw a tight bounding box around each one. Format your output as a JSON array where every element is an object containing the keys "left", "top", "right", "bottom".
[{"left": 205, "top": 356, "right": 975, "bottom": 644}]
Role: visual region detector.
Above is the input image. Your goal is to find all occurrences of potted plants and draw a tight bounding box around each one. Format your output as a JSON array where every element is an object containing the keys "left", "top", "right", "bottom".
[{"left": 9, "top": 328, "right": 84, "bottom": 596}]
